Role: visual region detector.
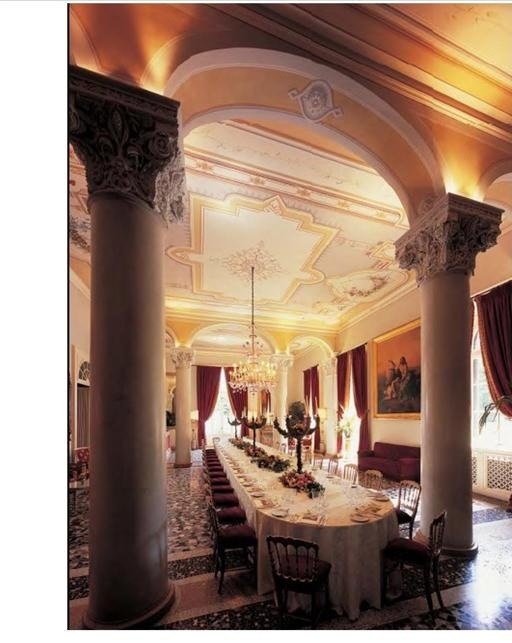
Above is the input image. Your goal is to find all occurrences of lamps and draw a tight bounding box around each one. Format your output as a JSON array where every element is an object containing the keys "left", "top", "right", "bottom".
[{"left": 228, "top": 266, "right": 281, "bottom": 395}]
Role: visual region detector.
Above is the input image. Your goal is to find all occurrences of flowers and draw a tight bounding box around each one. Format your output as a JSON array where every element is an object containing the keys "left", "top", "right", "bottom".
[
  {"left": 242, "top": 442, "right": 256, "bottom": 454},
  {"left": 282, "top": 464, "right": 310, "bottom": 490},
  {"left": 256, "top": 448, "right": 287, "bottom": 470}
]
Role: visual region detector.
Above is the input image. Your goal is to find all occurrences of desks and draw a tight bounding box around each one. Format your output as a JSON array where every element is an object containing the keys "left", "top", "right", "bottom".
[{"left": 202, "top": 441, "right": 396, "bottom": 532}]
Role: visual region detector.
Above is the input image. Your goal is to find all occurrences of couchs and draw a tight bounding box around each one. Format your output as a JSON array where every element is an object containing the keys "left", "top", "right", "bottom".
[{"left": 357, "top": 441, "right": 423, "bottom": 481}]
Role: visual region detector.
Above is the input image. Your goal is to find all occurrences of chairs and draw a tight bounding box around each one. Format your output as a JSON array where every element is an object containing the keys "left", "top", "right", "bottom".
[
  {"left": 266, "top": 537, "right": 330, "bottom": 625},
  {"left": 381, "top": 511, "right": 447, "bottom": 622},
  {"left": 201, "top": 440, "right": 449, "bottom": 501},
  {"left": 208, "top": 504, "right": 259, "bottom": 594},
  {"left": 395, "top": 477, "right": 423, "bottom": 540}
]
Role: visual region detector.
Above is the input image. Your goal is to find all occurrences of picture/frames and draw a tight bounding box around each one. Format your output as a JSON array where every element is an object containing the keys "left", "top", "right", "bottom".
[{"left": 370, "top": 317, "right": 420, "bottom": 421}]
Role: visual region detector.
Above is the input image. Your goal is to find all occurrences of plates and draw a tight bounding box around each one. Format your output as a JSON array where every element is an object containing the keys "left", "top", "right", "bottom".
[
  {"left": 272, "top": 509, "right": 289, "bottom": 517},
  {"left": 374, "top": 496, "right": 389, "bottom": 501},
  {"left": 230, "top": 464, "right": 266, "bottom": 498},
  {"left": 350, "top": 515, "right": 369, "bottom": 522}
]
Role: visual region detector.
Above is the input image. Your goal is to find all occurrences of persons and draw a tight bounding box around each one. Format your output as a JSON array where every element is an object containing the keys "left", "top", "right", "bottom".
[{"left": 382, "top": 356, "right": 411, "bottom": 400}]
